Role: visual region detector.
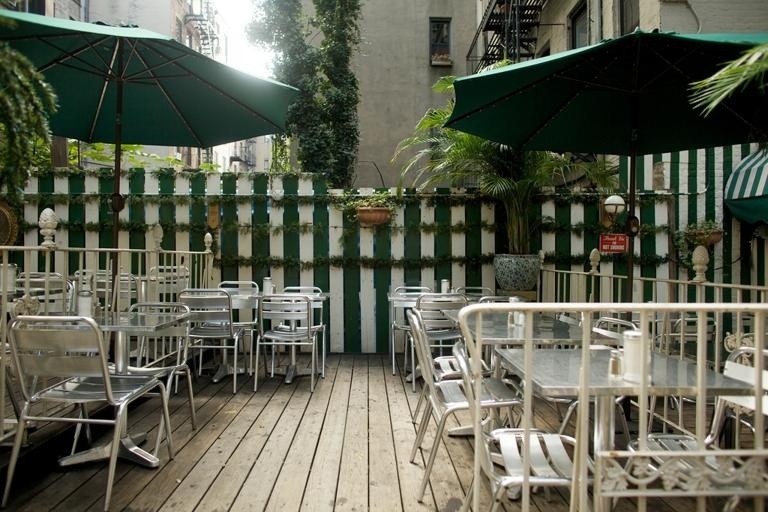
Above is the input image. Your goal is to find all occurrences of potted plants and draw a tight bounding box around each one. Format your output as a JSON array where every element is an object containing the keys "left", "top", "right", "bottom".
[
  {"left": 676, "top": 222, "right": 725, "bottom": 255},
  {"left": 390, "top": 58, "right": 612, "bottom": 291},
  {"left": 338, "top": 189, "right": 399, "bottom": 226}
]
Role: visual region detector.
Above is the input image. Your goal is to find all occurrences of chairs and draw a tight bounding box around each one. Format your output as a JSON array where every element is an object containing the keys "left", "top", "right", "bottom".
[
  {"left": 0, "top": 261, "right": 329, "bottom": 511},
  {"left": 391, "top": 285, "right": 768, "bottom": 511}
]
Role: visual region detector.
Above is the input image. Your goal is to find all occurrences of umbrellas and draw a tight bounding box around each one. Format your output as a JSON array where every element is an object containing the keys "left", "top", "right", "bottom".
[
  {"left": 0, "top": 5, "right": 303, "bottom": 363},
  {"left": 441, "top": 19, "right": 767, "bottom": 417}
]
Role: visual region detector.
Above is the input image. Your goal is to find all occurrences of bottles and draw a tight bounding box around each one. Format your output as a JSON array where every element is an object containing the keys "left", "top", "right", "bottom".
[
  {"left": 76, "top": 290, "right": 94, "bottom": 325},
  {"left": 1, "top": 262, "right": 17, "bottom": 295},
  {"left": 621, "top": 329, "right": 649, "bottom": 385},
  {"left": 507, "top": 295, "right": 525, "bottom": 328},
  {"left": 263, "top": 276, "right": 273, "bottom": 297},
  {"left": 607, "top": 349, "right": 626, "bottom": 375}
]
